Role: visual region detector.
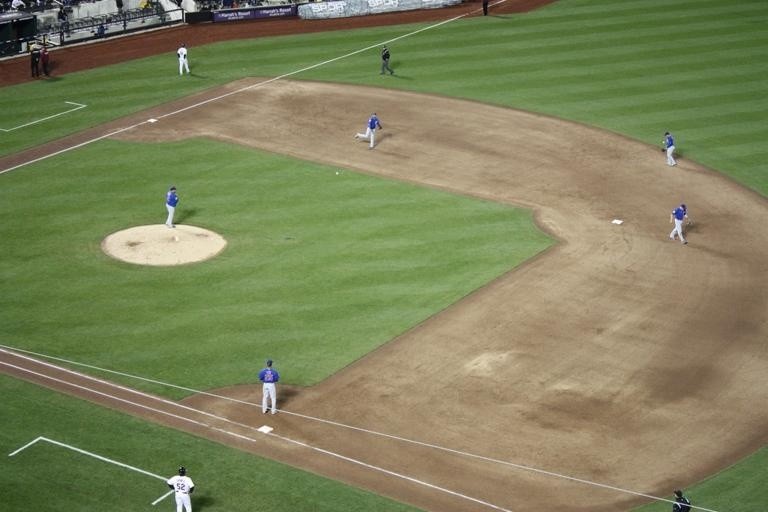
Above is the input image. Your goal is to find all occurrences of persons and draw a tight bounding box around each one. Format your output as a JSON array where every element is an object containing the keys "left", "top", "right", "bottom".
[
  {"left": 40, "top": 46, "right": 50, "bottom": 78},
  {"left": 116, "top": 0, "right": 123, "bottom": 12},
  {"left": 201, "top": 0, "right": 262, "bottom": 10},
  {"left": 258, "top": 359, "right": 279, "bottom": 416},
  {"left": 672, "top": 490, "right": 692, "bottom": 512},
  {"left": 662, "top": 131, "right": 678, "bottom": 167},
  {"left": 669, "top": 204, "right": 692, "bottom": 246},
  {"left": 165, "top": 187, "right": 179, "bottom": 229},
  {"left": 166, "top": 465, "right": 195, "bottom": 512},
  {"left": 353, "top": 113, "right": 383, "bottom": 150},
  {"left": 0, "top": 0, "right": 46, "bottom": 13},
  {"left": 176, "top": 43, "right": 191, "bottom": 76},
  {"left": 379, "top": 43, "right": 395, "bottom": 76},
  {"left": 482, "top": 0, "right": 489, "bottom": 16},
  {"left": 58, "top": 7, "right": 68, "bottom": 22},
  {"left": 31, "top": 44, "right": 41, "bottom": 78}
]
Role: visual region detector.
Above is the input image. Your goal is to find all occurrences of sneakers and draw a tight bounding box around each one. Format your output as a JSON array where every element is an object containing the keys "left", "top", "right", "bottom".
[
  {"left": 263, "top": 410, "right": 277, "bottom": 414},
  {"left": 669, "top": 235, "right": 688, "bottom": 244}
]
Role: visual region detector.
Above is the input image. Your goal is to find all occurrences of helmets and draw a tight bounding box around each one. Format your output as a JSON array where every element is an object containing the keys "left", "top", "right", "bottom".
[{"left": 179, "top": 467, "right": 186, "bottom": 474}]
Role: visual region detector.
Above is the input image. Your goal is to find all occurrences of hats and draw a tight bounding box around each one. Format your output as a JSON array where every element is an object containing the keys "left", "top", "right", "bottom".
[
  {"left": 267, "top": 360, "right": 273, "bottom": 366},
  {"left": 680, "top": 204, "right": 687, "bottom": 209}
]
